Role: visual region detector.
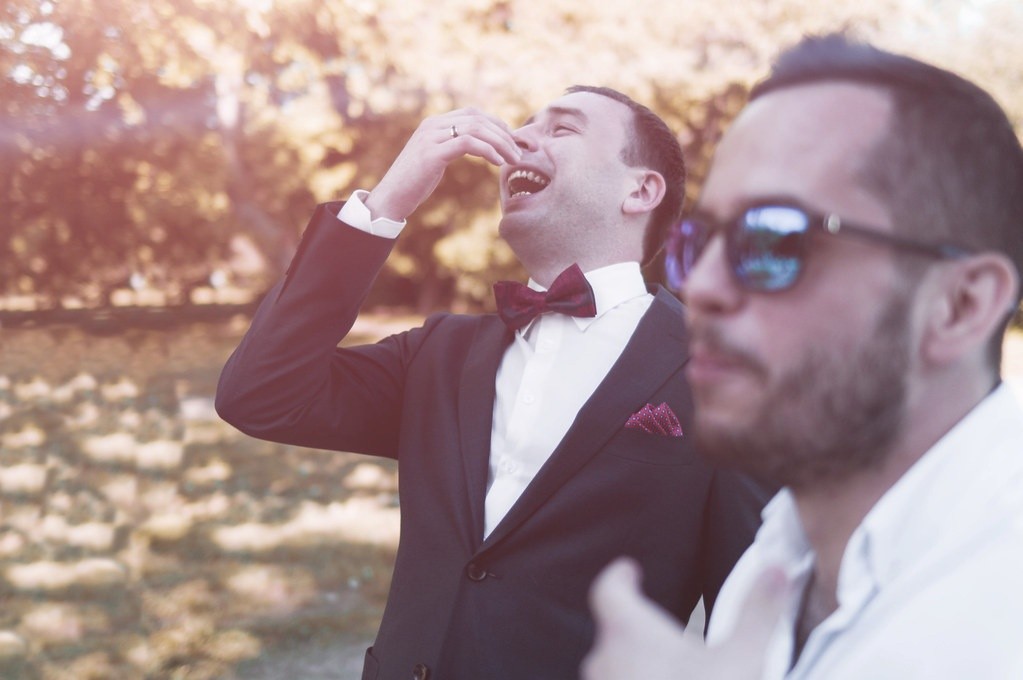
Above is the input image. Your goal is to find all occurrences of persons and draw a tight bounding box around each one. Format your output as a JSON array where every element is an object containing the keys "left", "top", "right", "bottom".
[
  {"left": 576, "top": 33, "right": 1023, "bottom": 680},
  {"left": 216, "top": 84, "right": 781, "bottom": 680}
]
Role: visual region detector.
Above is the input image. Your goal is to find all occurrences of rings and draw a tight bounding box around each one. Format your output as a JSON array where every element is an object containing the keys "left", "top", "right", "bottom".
[{"left": 451, "top": 126, "right": 458, "bottom": 137}]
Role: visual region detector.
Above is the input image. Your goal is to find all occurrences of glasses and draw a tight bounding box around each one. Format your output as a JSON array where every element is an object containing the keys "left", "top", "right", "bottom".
[{"left": 663, "top": 201, "right": 966, "bottom": 294}]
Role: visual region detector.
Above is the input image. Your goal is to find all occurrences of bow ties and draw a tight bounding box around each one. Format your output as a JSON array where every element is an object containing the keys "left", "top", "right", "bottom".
[{"left": 493, "top": 262, "right": 597, "bottom": 332}]
